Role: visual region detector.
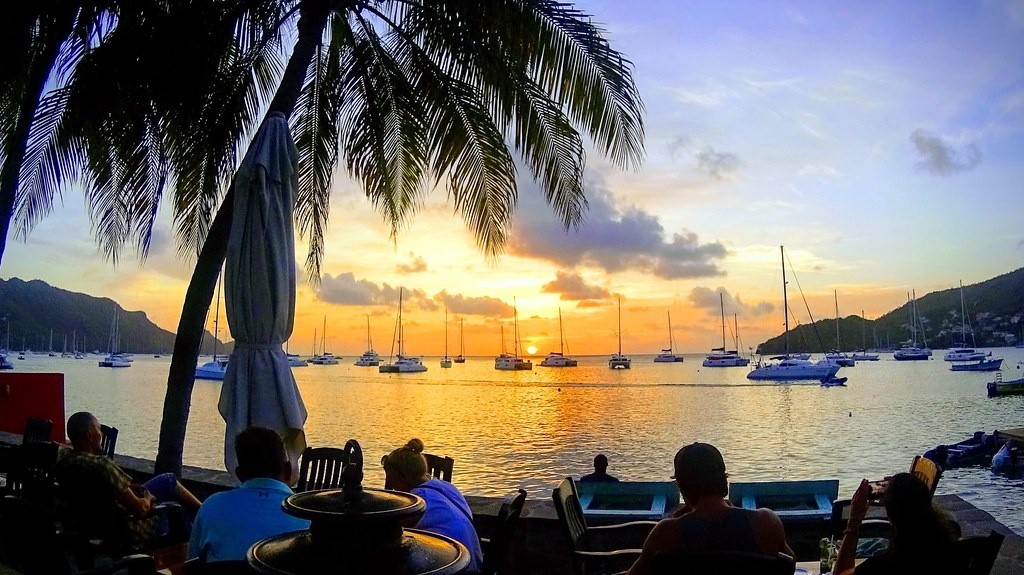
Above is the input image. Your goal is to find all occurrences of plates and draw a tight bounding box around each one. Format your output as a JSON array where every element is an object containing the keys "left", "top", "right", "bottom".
[{"left": 794, "top": 567, "right": 814, "bottom": 575}]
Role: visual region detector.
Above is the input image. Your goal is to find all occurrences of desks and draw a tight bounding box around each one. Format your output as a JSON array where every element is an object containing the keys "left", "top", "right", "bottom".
[{"left": 794, "top": 557, "right": 868, "bottom": 575}]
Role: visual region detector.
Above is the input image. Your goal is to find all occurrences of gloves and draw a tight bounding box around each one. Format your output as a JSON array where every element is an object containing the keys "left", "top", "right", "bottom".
[{"left": 848, "top": 478, "right": 874, "bottom": 523}]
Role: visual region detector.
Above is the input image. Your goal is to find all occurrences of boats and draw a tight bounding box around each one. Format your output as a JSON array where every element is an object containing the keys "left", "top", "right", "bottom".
[
  {"left": 153, "top": 355, "right": 163, "bottom": 358},
  {"left": 821, "top": 375, "right": 847, "bottom": 387},
  {"left": 778, "top": 354, "right": 812, "bottom": 362},
  {"left": 987, "top": 372, "right": 1024, "bottom": 398}
]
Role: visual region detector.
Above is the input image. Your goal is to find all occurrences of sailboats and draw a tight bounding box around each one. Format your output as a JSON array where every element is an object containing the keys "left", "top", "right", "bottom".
[
  {"left": 818, "top": 290, "right": 894, "bottom": 367},
  {"left": 195, "top": 265, "right": 230, "bottom": 380},
  {"left": 944, "top": 280, "right": 1004, "bottom": 371},
  {"left": 454, "top": 319, "right": 466, "bottom": 363},
  {"left": 495, "top": 297, "right": 533, "bottom": 370},
  {"left": 705, "top": 295, "right": 750, "bottom": 367},
  {"left": 609, "top": 298, "right": 632, "bottom": 369},
  {"left": 536, "top": 309, "right": 578, "bottom": 368},
  {"left": 1016, "top": 325, "right": 1024, "bottom": 348},
  {"left": 354, "top": 286, "right": 428, "bottom": 373},
  {"left": 748, "top": 252, "right": 841, "bottom": 380},
  {"left": 440, "top": 311, "right": 452, "bottom": 369},
  {"left": 894, "top": 291, "right": 933, "bottom": 361},
  {"left": 654, "top": 314, "right": 683, "bottom": 364},
  {"left": 285, "top": 314, "right": 344, "bottom": 366},
  {"left": 98, "top": 312, "right": 134, "bottom": 371},
  {"left": 0, "top": 316, "right": 100, "bottom": 372}
]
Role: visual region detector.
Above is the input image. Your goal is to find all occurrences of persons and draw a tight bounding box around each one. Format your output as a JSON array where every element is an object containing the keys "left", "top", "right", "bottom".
[
  {"left": 381, "top": 439, "right": 483, "bottom": 575},
  {"left": 833, "top": 473, "right": 960, "bottom": 575},
  {"left": 188, "top": 427, "right": 310, "bottom": 562},
  {"left": 48, "top": 413, "right": 203, "bottom": 550},
  {"left": 626, "top": 442, "right": 795, "bottom": 575},
  {"left": 580, "top": 454, "right": 619, "bottom": 483}
]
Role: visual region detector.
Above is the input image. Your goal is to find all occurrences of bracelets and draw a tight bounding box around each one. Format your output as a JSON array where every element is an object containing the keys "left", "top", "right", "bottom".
[{"left": 843, "top": 518, "right": 861, "bottom": 536}]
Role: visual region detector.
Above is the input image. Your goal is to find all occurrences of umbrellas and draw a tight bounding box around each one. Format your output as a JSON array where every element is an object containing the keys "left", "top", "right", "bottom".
[{"left": 218, "top": 112, "right": 308, "bottom": 483}]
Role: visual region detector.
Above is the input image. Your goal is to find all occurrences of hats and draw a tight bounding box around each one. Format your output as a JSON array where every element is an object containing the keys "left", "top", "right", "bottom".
[{"left": 670, "top": 441, "right": 726, "bottom": 484}]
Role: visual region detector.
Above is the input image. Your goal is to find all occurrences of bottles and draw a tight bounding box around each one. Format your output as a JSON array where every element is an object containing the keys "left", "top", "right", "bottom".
[{"left": 820, "top": 544, "right": 838, "bottom": 575}]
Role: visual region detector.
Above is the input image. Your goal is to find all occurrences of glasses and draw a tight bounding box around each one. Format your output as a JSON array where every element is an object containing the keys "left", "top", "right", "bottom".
[{"left": 380, "top": 455, "right": 405, "bottom": 480}]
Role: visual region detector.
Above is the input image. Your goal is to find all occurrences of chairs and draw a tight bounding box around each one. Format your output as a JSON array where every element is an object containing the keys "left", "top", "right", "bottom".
[
  {"left": 866, "top": 529, "right": 1005, "bottom": 575},
  {"left": 0, "top": 418, "right": 526, "bottom": 575},
  {"left": 552, "top": 475, "right": 657, "bottom": 575},
  {"left": 832, "top": 455, "right": 942, "bottom": 544}
]
